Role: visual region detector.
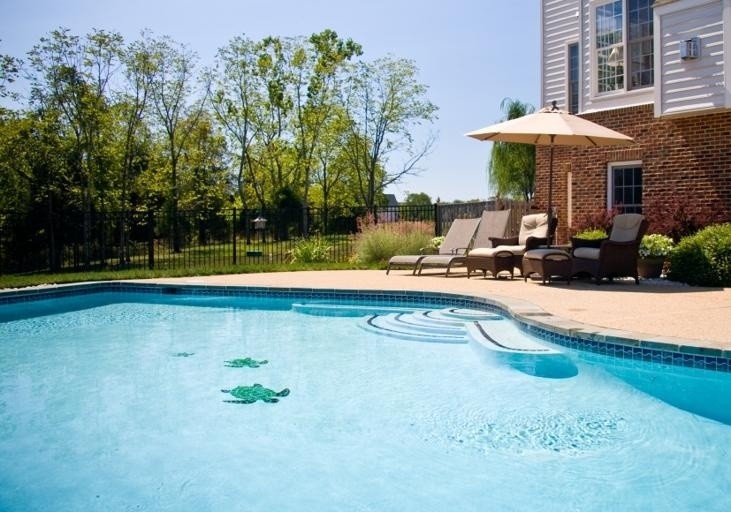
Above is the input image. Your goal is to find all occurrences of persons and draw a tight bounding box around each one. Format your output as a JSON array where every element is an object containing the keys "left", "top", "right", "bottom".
[{"left": 250, "top": 216, "right": 267, "bottom": 242}]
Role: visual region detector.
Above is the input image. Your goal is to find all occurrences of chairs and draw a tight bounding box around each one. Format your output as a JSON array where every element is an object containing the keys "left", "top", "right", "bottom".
[
  {"left": 417, "top": 209, "right": 513, "bottom": 277},
  {"left": 386, "top": 217, "right": 481, "bottom": 275},
  {"left": 487, "top": 213, "right": 558, "bottom": 276},
  {"left": 569, "top": 213, "right": 648, "bottom": 286}
]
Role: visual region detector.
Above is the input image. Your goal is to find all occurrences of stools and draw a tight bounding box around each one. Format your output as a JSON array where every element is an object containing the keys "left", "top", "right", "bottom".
[
  {"left": 522, "top": 249, "right": 574, "bottom": 286},
  {"left": 466, "top": 247, "right": 516, "bottom": 280}
]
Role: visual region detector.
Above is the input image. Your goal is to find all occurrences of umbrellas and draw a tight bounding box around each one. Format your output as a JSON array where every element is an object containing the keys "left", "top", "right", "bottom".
[{"left": 464, "top": 100, "right": 638, "bottom": 247}]
